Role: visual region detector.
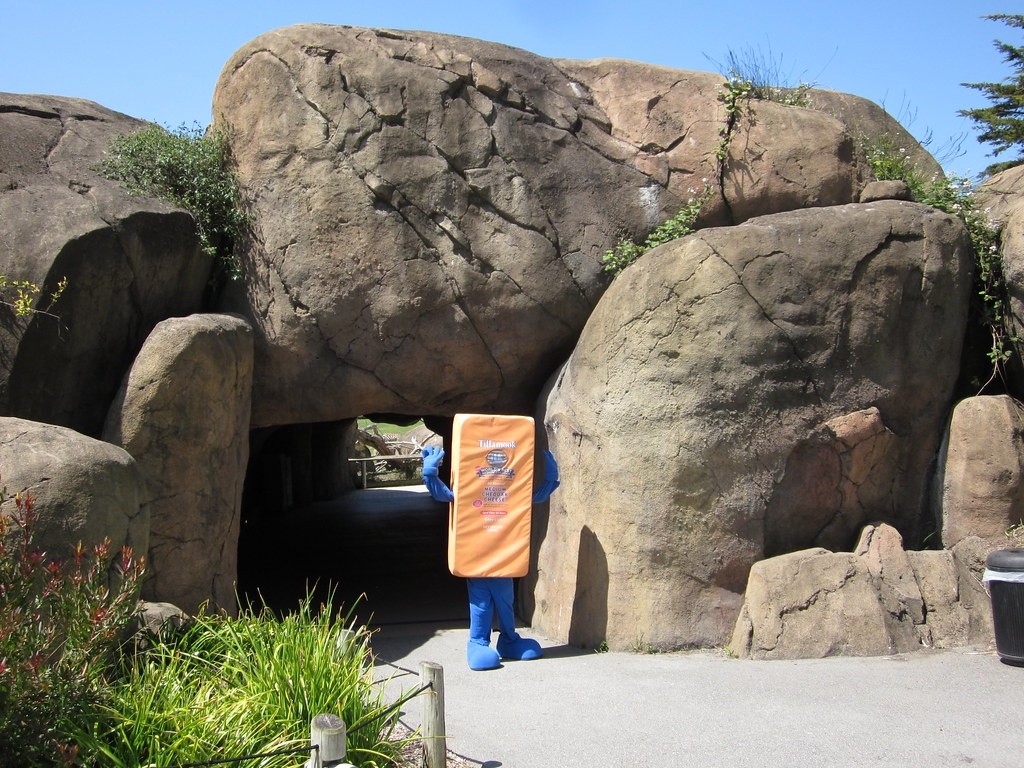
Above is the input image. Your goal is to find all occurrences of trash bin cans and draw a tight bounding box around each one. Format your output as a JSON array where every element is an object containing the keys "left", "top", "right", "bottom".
[{"left": 984, "top": 548, "right": 1024, "bottom": 668}]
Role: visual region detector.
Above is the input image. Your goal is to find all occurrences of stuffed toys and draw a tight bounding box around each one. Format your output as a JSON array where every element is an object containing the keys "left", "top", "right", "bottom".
[{"left": 422, "top": 415, "right": 559, "bottom": 671}]
